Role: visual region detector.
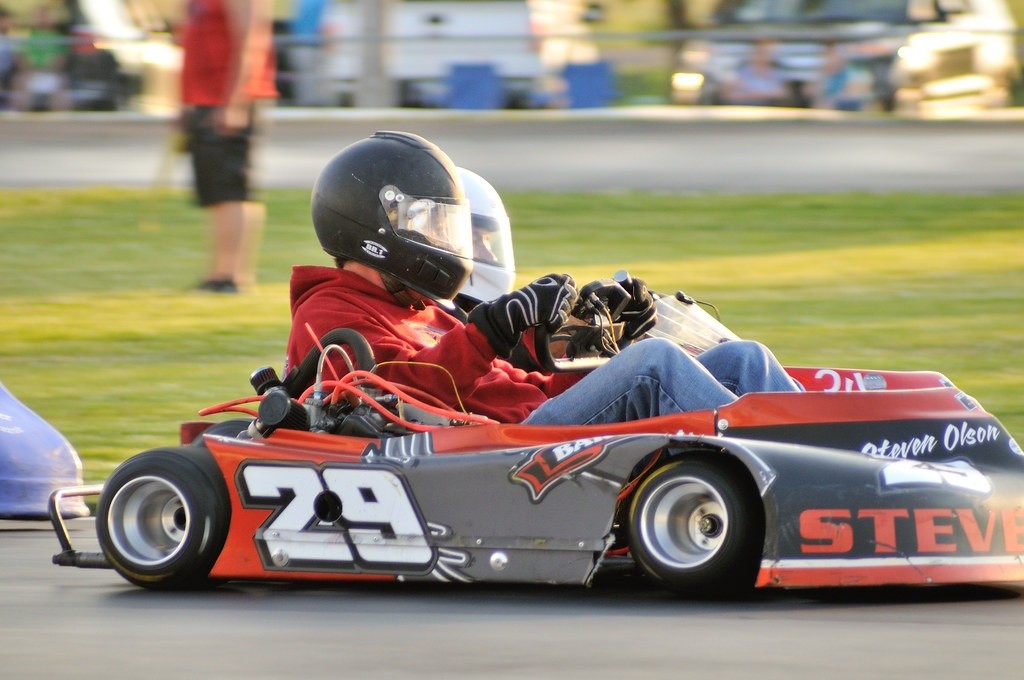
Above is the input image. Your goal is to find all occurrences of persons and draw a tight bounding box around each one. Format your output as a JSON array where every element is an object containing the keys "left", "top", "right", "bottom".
[
  {"left": 0, "top": 0, "right": 900, "bottom": 299},
  {"left": 281, "top": 129, "right": 809, "bottom": 424}
]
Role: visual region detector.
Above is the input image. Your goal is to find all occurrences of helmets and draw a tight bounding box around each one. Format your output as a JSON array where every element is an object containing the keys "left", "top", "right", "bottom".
[
  {"left": 312, "top": 131, "right": 473, "bottom": 298},
  {"left": 447, "top": 162, "right": 516, "bottom": 304}
]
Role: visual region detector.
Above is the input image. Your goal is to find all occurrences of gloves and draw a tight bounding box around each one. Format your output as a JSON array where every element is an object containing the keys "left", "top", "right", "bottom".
[
  {"left": 488, "top": 273, "right": 578, "bottom": 344},
  {"left": 572, "top": 276, "right": 658, "bottom": 339}
]
